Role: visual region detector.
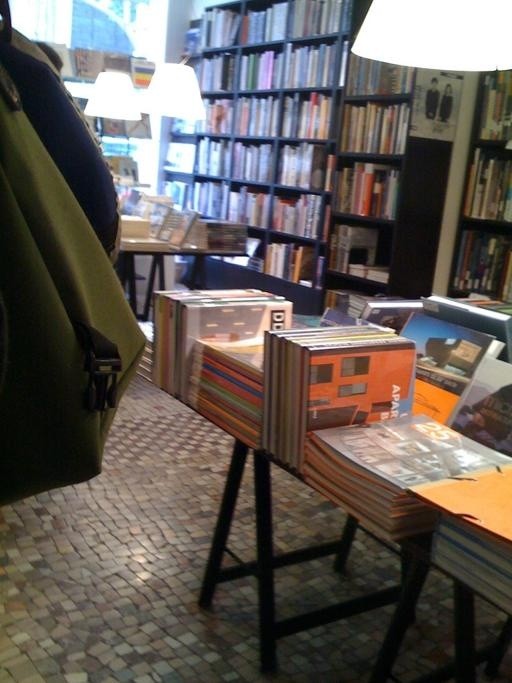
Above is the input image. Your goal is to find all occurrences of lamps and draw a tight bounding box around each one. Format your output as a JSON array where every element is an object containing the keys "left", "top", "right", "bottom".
[{"left": 83, "top": 63, "right": 206, "bottom": 120}]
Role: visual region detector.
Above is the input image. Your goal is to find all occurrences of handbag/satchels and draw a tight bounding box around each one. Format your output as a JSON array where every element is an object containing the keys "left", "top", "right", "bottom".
[{"left": 1, "top": 74, "right": 149, "bottom": 508}]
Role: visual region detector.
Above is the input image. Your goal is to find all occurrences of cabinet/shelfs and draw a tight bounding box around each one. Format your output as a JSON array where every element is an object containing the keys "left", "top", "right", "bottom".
[{"left": 163, "top": 0, "right": 512, "bottom": 316}]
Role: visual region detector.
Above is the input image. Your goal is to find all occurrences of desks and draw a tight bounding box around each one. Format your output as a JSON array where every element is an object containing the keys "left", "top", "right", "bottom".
[{"left": 134, "top": 322, "right": 512, "bottom": 683}]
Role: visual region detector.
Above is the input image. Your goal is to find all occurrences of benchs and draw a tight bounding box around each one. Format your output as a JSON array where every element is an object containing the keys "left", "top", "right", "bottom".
[{"left": 117, "top": 251, "right": 250, "bottom": 321}]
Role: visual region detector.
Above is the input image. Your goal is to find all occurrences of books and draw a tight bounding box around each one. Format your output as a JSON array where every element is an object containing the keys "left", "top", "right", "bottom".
[
  {"left": 156, "top": 288, "right": 418, "bottom": 470},
  {"left": 398, "top": 293, "right": 512, "bottom": 455},
  {"left": 301, "top": 413, "right": 511, "bottom": 615},
  {"left": 163, "top": 2, "right": 353, "bottom": 291},
  {"left": 348, "top": 53, "right": 415, "bottom": 284},
  {"left": 452, "top": 70, "right": 511, "bottom": 316}
]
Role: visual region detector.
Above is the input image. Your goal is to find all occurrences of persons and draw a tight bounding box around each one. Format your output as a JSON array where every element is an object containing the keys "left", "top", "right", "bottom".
[
  {"left": 436, "top": 83, "right": 453, "bottom": 128},
  {"left": 423, "top": 77, "right": 440, "bottom": 130}
]
